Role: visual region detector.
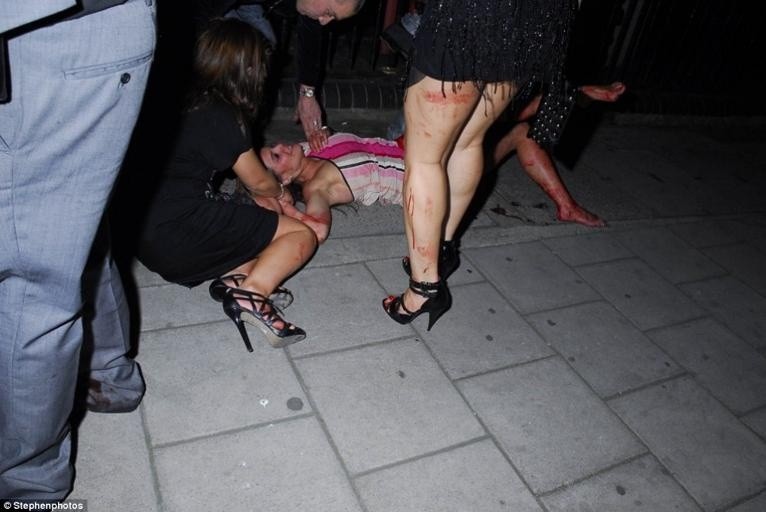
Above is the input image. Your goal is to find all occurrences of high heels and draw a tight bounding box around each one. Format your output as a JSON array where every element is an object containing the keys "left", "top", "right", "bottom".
[
  {"left": 210, "top": 275, "right": 307, "bottom": 352},
  {"left": 380, "top": 279, "right": 451, "bottom": 329},
  {"left": 401, "top": 239, "right": 459, "bottom": 282}
]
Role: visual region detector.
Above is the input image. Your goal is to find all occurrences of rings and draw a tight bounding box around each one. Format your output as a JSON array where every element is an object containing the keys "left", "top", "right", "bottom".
[
  {"left": 321, "top": 126, "right": 328, "bottom": 130},
  {"left": 313, "top": 121, "right": 317, "bottom": 126}
]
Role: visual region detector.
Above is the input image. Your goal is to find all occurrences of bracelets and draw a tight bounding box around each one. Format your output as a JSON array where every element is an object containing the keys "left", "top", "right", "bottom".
[
  {"left": 298, "top": 87, "right": 315, "bottom": 100},
  {"left": 276, "top": 184, "right": 284, "bottom": 200}
]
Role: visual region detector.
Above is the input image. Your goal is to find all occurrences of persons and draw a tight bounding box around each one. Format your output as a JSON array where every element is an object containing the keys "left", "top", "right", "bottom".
[
  {"left": 0, "top": 0, "right": 163, "bottom": 511},
  {"left": 177, "top": 1, "right": 369, "bottom": 140},
  {"left": 114, "top": 18, "right": 318, "bottom": 352},
  {"left": 382, "top": 1, "right": 532, "bottom": 331},
  {"left": 253, "top": 72, "right": 627, "bottom": 242}
]
